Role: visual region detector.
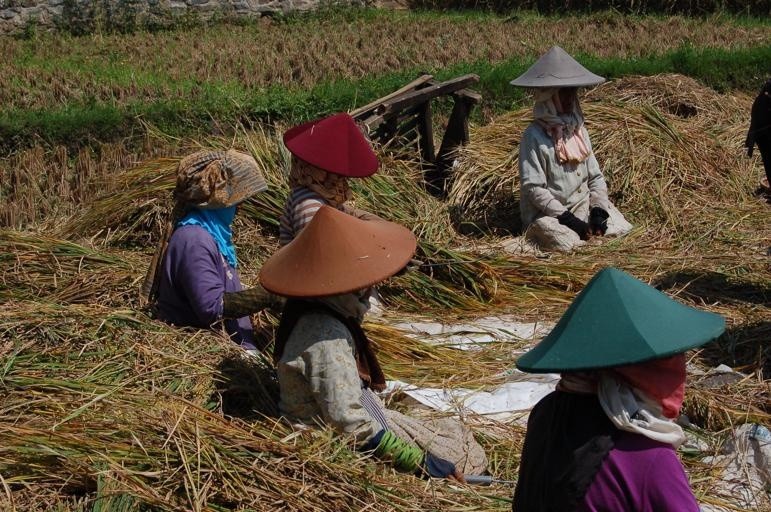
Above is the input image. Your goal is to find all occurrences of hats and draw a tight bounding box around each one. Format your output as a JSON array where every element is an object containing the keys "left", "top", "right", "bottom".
[
  {"left": 258, "top": 203, "right": 418, "bottom": 298},
  {"left": 509, "top": 43, "right": 606, "bottom": 90},
  {"left": 283, "top": 111, "right": 380, "bottom": 179},
  {"left": 515, "top": 265, "right": 727, "bottom": 374}
]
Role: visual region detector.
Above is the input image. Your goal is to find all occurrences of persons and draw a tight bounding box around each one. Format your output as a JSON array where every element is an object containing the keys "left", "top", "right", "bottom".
[
  {"left": 283, "top": 113, "right": 382, "bottom": 313},
  {"left": 513, "top": 268, "right": 727, "bottom": 512},
  {"left": 260, "top": 206, "right": 488, "bottom": 483},
  {"left": 143, "top": 151, "right": 287, "bottom": 376},
  {"left": 509, "top": 44, "right": 634, "bottom": 251}
]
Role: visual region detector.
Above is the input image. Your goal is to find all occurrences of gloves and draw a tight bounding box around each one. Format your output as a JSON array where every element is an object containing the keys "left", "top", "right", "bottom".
[
  {"left": 588, "top": 206, "right": 610, "bottom": 237},
  {"left": 556, "top": 211, "right": 590, "bottom": 241}
]
